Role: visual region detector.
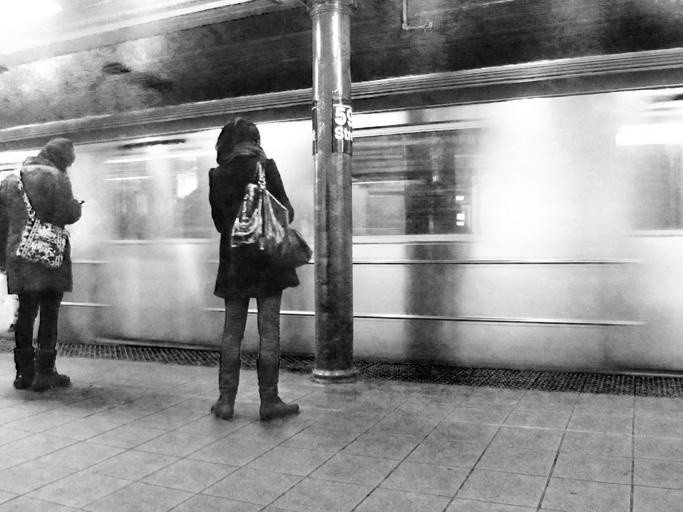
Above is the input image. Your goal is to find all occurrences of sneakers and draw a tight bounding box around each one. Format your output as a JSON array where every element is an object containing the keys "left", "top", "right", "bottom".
[{"left": 13, "top": 345, "right": 69, "bottom": 392}]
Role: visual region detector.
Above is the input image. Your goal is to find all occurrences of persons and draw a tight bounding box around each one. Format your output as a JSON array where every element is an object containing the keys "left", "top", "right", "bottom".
[
  {"left": 208, "top": 115, "right": 300, "bottom": 424},
  {"left": 0, "top": 136, "right": 87, "bottom": 395}
]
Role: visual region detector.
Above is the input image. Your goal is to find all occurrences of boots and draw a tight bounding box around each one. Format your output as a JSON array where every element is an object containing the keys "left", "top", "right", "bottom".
[
  {"left": 255, "top": 359, "right": 298, "bottom": 421},
  {"left": 210, "top": 356, "right": 242, "bottom": 419}
]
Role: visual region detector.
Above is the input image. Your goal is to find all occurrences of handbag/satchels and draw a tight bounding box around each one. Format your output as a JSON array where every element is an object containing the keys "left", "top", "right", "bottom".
[
  {"left": 15, "top": 216, "right": 69, "bottom": 270},
  {"left": 229, "top": 184, "right": 311, "bottom": 269}
]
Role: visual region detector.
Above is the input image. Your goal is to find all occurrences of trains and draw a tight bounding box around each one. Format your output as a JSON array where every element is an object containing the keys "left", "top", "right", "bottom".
[{"left": 0, "top": 47, "right": 682, "bottom": 383}]
